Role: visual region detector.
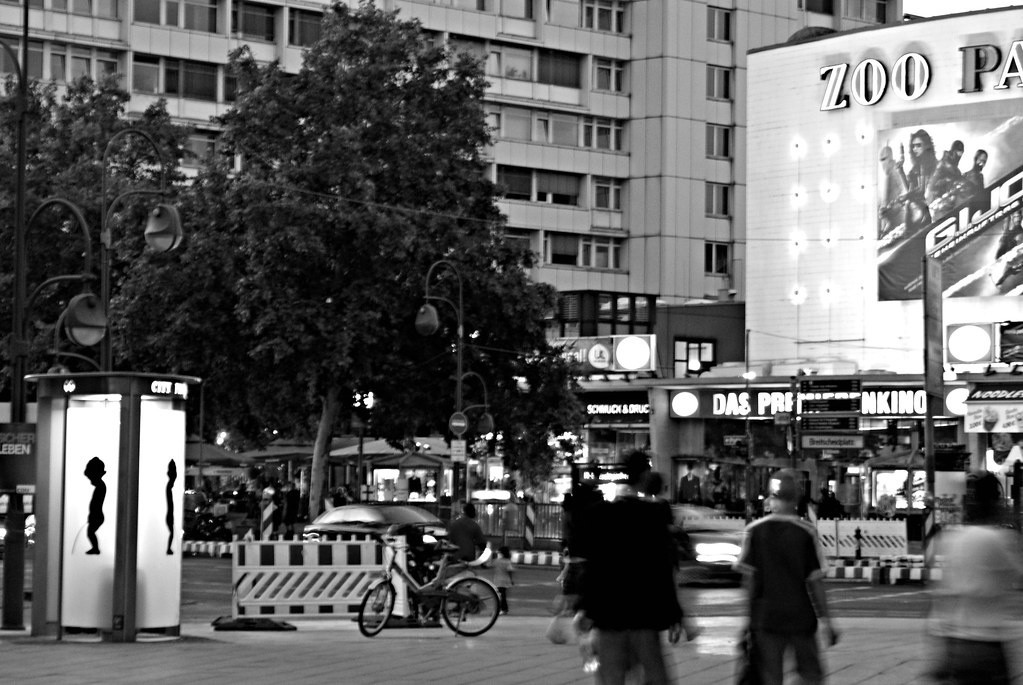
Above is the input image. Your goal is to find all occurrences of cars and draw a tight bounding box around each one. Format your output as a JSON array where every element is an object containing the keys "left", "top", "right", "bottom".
[
  {"left": 676, "top": 530, "right": 748, "bottom": 587},
  {"left": 301, "top": 495, "right": 450, "bottom": 573}
]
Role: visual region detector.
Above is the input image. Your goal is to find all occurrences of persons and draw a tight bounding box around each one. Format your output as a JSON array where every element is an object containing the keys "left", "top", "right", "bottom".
[
  {"left": 878, "top": 129, "right": 988, "bottom": 240},
  {"left": 183, "top": 475, "right": 298, "bottom": 540},
  {"left": 740, "top": 471, "right": 838, "bottom": 684},
  {"left": 550, "top": 450, "right": 685, "bottom": 684},
  {"left": 677, "top": 460, "right": 700, "bottom": 503},
  {"left": 449, "top": 501, "right": 487, "bottom": 562},
  {"left": 921, "top": 469, "right": 1023, "bottom": 685}
]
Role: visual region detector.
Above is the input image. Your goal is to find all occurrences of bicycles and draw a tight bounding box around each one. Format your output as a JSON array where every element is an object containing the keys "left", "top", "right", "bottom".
[{"left": 355, "top": 533, "right": 502, "bottom": 637}]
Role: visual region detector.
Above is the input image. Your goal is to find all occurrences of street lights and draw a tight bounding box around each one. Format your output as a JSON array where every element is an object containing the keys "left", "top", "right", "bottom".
[
  {"left": 49, "top": 128, "right": 185, "bottom": 378},
  {"left": 413, "top": 260, "right": 495, "bottom": 528},
  {"left": 1, "top": 18, "right": 107, "bottom": 629}
]
